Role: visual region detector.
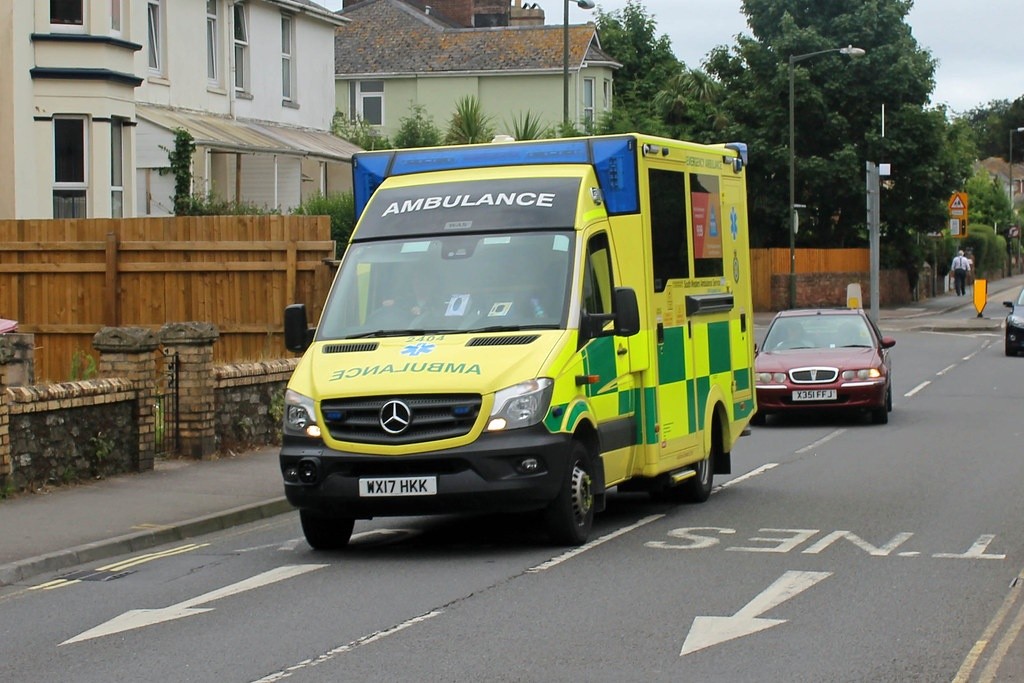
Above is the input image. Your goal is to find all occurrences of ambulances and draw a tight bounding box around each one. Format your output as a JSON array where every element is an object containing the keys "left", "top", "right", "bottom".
[{"left": 279, "top": 133, "right": 758, "bottom": 550}]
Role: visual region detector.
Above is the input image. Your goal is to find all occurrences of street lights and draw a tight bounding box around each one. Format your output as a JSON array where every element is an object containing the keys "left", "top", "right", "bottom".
[
  {"left": 1009, "top": 127, "right": 1024, "bottom": 199},
  {"left": 790, "top": 45, "right": 865, "bottom": 308},
  {"left": 564, "top": 0, "right": 595, "bottom": 126}
]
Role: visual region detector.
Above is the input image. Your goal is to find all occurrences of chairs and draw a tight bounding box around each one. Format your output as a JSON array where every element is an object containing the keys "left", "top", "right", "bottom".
[{"left": 836, "top": 324, "right": 861, "bottom": 345}]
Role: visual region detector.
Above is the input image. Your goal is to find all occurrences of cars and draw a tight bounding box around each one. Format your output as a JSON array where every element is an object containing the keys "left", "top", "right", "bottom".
[
  {"left": 750, "top": 308, "right": 892, "bottom": 426},
  {"left": 1002, "top": 287, "right": 1024, "bottom": 356}
]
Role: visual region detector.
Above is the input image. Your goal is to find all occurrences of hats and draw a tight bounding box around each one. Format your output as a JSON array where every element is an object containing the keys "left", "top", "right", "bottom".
[{"left": 957, "top": 250, "right": 964, "bottom": 256}]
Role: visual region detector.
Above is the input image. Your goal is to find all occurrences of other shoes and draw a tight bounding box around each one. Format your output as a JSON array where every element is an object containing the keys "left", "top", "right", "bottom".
[{"left": 963, "top": 292, "right": 966, "bottom": 295}]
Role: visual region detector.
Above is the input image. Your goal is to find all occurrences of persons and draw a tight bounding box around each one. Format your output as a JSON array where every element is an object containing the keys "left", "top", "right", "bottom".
[
  {"left": 949, "top": 250, "right": 971, "bottom": 296},
  {"left": 381, "top": 262, "right": 446, "bottom": 329},
  {"left": 532, "top": 261, "right": 589, "bottom": 320},
  {"left": 908, "top": 261, "right": 919, "bottom": 302},
  {"left": 776, "top": 321, "right": 818, "bottom": 350}
]
